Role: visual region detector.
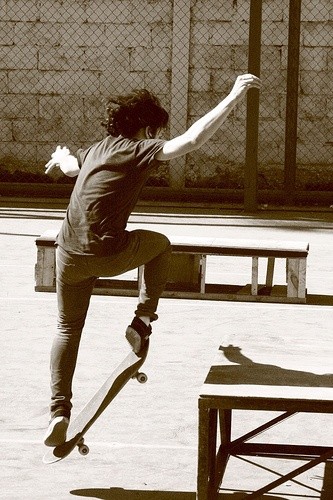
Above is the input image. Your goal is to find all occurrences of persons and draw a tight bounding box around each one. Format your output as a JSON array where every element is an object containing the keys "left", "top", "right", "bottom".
[{"left": 43, "top": 74, "right": 262, "bottom": 447}]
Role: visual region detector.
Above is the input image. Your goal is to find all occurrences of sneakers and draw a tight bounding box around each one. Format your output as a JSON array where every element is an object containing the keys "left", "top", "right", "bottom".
[
  {"left": 126, "top": 317, "right": 152, "bottom": 353},
  {"left": 45, "top": 406, "right": 70, "bottom": 446}
]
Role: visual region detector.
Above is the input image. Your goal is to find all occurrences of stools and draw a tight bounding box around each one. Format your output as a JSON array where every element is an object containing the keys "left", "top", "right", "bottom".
[{"left": 195, "top": 362, "right": 332, "bottom": 499}]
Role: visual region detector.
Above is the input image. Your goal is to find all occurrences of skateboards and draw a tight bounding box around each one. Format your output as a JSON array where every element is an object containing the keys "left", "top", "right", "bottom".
[{"left": 42, "top": 340, "right": 148, "bottom": 464}]
